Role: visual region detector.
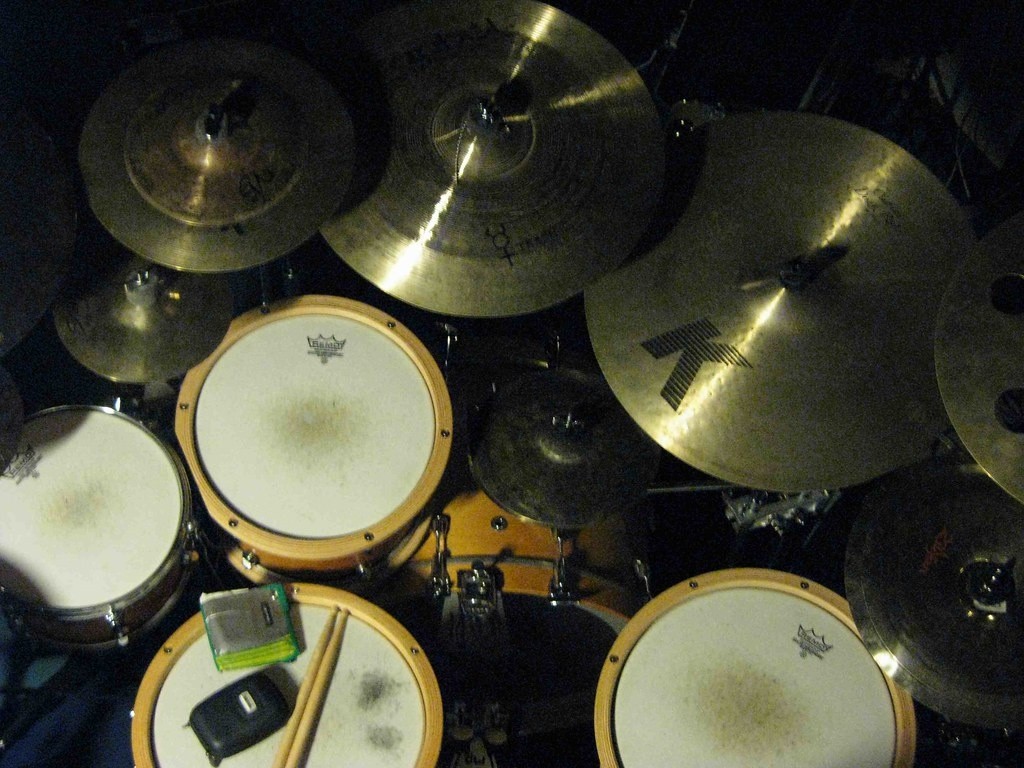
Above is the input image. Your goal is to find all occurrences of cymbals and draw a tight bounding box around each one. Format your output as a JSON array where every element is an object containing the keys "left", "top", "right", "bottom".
[
  {"left": 0, "top": 96, "right": 78, "bottom": 361},
  {"left": 843, "top": 435, "right": 1024, "bottom": 732},
  {"left": 51, "top": 233, "right": 233, "bottom": 383},
  {"left": 584, "top": 107, "right": 980, "bottom": 493},
  {"left": 79, "top": 40, "right": 354, "bottom": 274},
  {"left": 318, "top": 1, "right": 664, "bottom": 320},
  {"left": 934, "top": 209, "right": 1024, "bottom": 505},
  {"left": 464, "top": 355, "right": 655, "bottom": 530},
  {"left": 0, "top": 365, "right": 24, "bottom": 475}
]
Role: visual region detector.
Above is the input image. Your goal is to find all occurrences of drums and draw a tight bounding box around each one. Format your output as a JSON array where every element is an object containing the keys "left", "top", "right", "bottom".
[
  {"left": 0, "top": 404, "right": 195, "bottom": 655},
  {"left": 365, "top": 474, "right": 638, "bottom": 736},
  {"left": 166, "top": 292, "right": 450, "bottom": 585},
  {"left": 596, "top": 569, "right": 917, "bottom": 768},
  {"left": 129, "top": 583, "right": 443, "bottom": 768}
]
